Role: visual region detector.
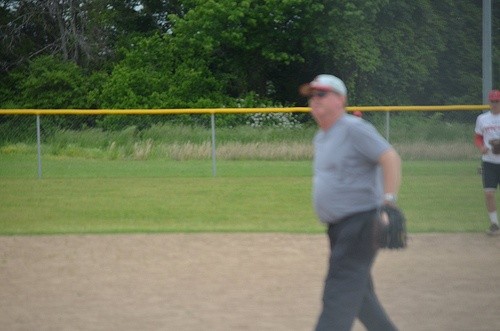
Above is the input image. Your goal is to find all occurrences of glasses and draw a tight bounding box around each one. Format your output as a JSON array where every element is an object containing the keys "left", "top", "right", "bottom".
[{"left": 306, "top": 93, "right": 340, "bottom": 98}]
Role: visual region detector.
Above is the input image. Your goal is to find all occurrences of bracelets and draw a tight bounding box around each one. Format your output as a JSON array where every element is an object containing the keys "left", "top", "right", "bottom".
[{"left": 385, "top": 192, "right": 398, "bottom": 202}]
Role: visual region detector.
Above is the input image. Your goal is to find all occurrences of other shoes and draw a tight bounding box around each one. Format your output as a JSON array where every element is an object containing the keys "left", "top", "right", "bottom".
[{"left": 488, "top": 223, "right": 500, "bottom": 235}]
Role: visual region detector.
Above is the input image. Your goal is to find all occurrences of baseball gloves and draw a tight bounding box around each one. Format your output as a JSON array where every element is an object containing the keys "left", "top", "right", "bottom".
[{"left": 368, "top": 206, "right": 409, "bottom": 250}]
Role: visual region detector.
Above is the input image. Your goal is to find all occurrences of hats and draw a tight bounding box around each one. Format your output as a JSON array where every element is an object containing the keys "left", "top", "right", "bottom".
[
  {"left": 488, "top": 90, "right": 500, "bottom": 101},
  {"left": 299, "top": 74, "right": 347, "bottom": 98}
]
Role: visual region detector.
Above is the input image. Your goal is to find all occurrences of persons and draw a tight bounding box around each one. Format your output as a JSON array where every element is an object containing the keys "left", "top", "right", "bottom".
[
  {"left": 299, "top": 74, "right": 407, "bottom": 331},
  {"left": 473, "top": 90, "right": 500, "bottom": 237}
]
